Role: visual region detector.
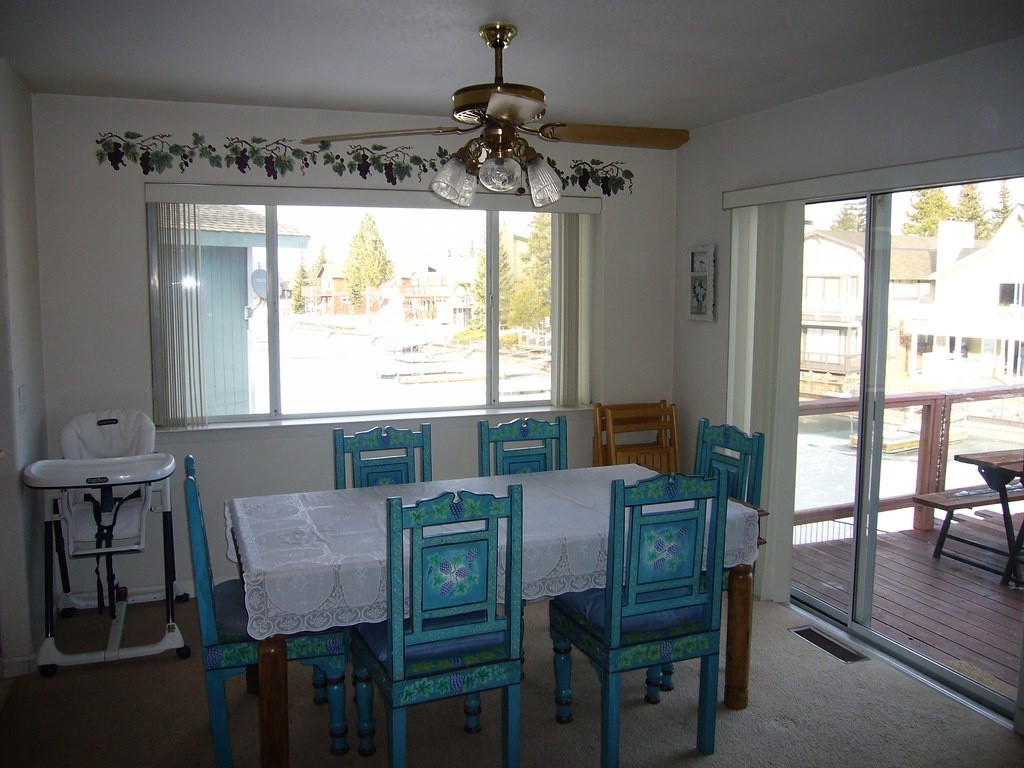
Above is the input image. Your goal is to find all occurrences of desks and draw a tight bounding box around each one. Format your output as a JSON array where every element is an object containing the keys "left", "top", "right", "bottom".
[
  {"left": 22, "top": 452, "right": 177, "bottom": 500},
  {"left": 954, "top": 449, "right": 1024, "bottom": 587},
  {"left": 222, "top": 465, "right": 770, "bottom": 768}
]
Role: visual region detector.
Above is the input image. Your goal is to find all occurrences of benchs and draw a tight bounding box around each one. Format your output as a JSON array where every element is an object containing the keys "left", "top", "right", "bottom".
[{"left": 912, "top": 479, "right": 1024, "bottom": 559}]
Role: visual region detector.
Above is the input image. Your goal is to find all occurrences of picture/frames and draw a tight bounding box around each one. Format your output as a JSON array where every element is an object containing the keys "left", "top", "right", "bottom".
[{"left": 686, "top": 245, "right": 715, "bottom": 322}]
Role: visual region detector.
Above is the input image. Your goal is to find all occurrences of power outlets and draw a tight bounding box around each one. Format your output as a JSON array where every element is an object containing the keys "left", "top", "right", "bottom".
[{"left": 19, "top": 385, "right": 29, "bottom": 413}]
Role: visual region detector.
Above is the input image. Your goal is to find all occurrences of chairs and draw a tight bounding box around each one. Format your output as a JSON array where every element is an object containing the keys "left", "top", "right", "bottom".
[
  {"left": 592, "top": 400, "right": 667, "bottom": 467},
  {"left": 548, "top": 472, "right": 729, "bottom": 768},
  {"left": 693, "top": 417, "right": 765, "bottom": 507},
  {"left": 333, "top": 423, "right": 432, "bottom": 489},
  {"left": 60, "top": 408, "right": 155, "bottom": 558},
  {"left": 605, "top": 404, "right": 682, "bottom": 473},
  {"left": 477, "top": 416, "right": 568, "bottom": 476},
  {"left": 351, "top": 483, "right": 523, "bottom": 655},
  {"left": 184, "top": 454, "right": 348, "bottom": 768}
]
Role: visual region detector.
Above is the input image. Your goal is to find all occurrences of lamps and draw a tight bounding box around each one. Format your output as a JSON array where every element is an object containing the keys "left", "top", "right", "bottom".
[
  {"left": 244, "top": 268, "right": 267, "bottom": 319},
  {"left": 431, "top": 127, "right": 562, "bottom": 208}
]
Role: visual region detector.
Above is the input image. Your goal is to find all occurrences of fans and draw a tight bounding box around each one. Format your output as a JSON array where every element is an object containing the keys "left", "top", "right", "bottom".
[{"left": 299, "top": 23, "right": 690, "bottom": 151}]
